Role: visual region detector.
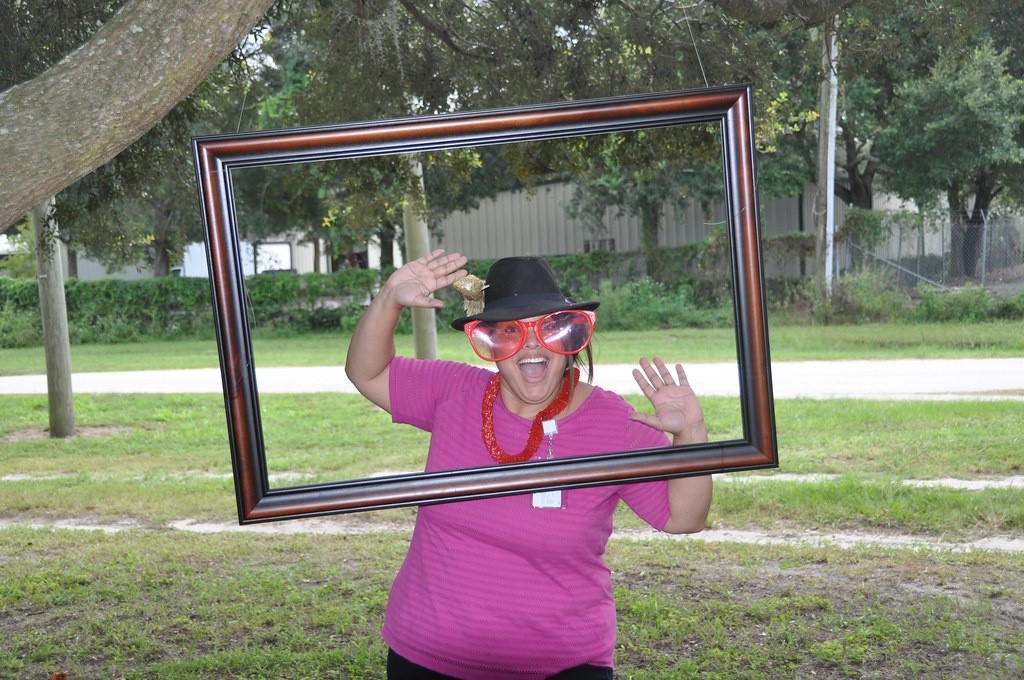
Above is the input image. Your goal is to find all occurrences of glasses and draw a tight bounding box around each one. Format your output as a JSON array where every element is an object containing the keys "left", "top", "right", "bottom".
[{"left": 464, "top": 309, "right": 597, "bottom": 362}]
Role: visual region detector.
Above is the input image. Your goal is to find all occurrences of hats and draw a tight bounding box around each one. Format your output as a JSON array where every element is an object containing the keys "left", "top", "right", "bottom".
[{"left": 450, "top": 256, "right": 600, "bottom": 331}]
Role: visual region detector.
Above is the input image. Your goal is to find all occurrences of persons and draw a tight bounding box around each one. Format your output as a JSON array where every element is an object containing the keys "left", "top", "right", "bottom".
[{"left": 345, "top": 249, "right": 712, "bottom": 680}]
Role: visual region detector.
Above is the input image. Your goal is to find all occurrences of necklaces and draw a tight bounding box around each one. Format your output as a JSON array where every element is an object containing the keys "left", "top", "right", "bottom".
[{"left": 481, "top": 367, "right": 580, "bottom": 464}]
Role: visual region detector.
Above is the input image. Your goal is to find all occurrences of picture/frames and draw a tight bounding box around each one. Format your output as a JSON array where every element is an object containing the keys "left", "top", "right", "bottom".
[{"left": 190, "top": 86, "right": 780, "bottom": 526}]
